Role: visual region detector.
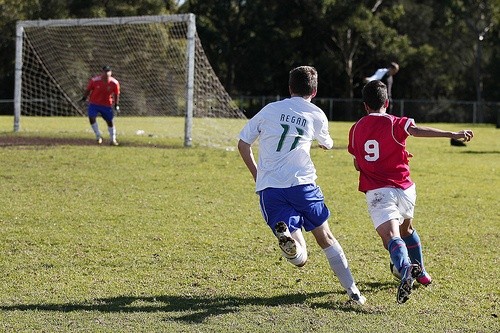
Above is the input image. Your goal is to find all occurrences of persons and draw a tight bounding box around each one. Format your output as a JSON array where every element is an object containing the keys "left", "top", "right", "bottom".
[
  {"left": 79, "top": 65, "right": 122, "bottom": 147},
  {"left": 347, "top": 79, "right": 475, "bottom": 305},
  {"left": 237, "top": 64, "right": 368, "bottom": 307},
  {"left": 363, "top": 62, "right": 400, "bottom": 101}
]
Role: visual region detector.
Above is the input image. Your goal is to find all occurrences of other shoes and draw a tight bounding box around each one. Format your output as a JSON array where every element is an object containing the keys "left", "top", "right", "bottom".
[
  {"left": 97, "top": 138, "right": 103, "bottom": 144},
  {"left": 274, "top": 221, "right": 300, "bottom": 259},
  {"left": 110, "top": 142, "right": 120, "bottom": 146},
  {"left": 346, "top": 288, "right": 368, "bottom": 306}
]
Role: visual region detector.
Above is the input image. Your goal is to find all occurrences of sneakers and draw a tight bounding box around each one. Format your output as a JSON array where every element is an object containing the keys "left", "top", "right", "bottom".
[
  {"left": 390, "top": 262, "right": 432, "bottom": 286},
  {"left": 397, "top": 264, "right": 422, "bottom": 303}
]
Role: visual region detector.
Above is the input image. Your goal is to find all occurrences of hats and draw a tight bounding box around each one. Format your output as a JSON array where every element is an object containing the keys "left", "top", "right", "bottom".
[{"left": 103, "top": 65, "right": 112, "bottom": 71}]
